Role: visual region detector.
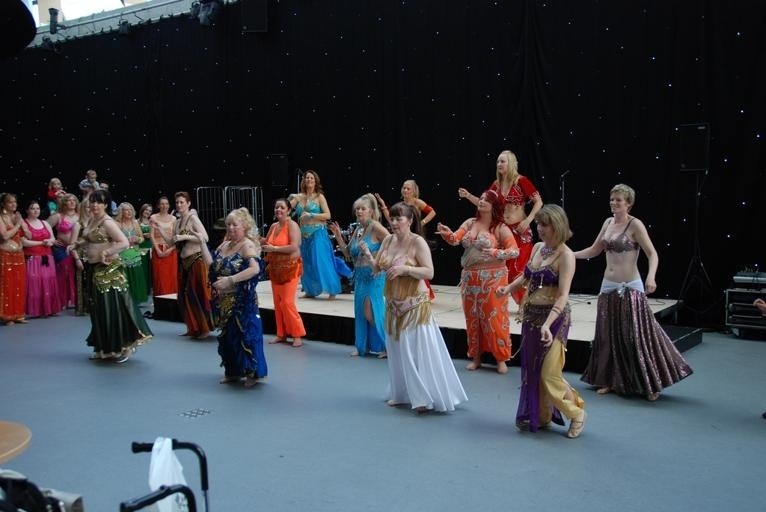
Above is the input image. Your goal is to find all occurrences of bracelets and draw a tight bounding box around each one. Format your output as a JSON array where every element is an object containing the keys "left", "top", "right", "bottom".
[
  {"left": 227, "top": 276, "right": 235, "bottom": 287},
  {"left": 421, "top": 219, "right": 426, "bottom": 225},
  {"left": 551, "top": 305, "right": 564, "bottom": 317},
  {"left": 74, "top": 257, "right": 81, "bottom": 261}
]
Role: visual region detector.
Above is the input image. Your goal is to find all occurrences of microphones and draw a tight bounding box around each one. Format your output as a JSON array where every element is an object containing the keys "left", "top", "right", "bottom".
[
  {"left": 296, "top": 168, "right": 304, "bottom": 176},
  {"left": 561, "top": 170, "right": 570, "bottom": 178}
]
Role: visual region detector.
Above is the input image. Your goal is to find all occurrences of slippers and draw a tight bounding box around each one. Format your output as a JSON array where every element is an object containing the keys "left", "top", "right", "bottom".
[
  {"left": 143, "top": 311, "right": 150, "bottom": 317},
  {"left": 148, "top": 313, "right": 154, "bottom": 318}
]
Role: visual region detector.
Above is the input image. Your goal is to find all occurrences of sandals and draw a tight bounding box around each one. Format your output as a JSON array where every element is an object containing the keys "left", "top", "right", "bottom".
[
  {"left": 351, "top": 349, "right": 368, "bottom": 356},
  {"left": 647, "top": 393, "right": 660, "bottom": 401},
  {"left": 377, "top": 352, "right": 387, "bottom": 359},
  {"left": 244, "top": 380, "right": 257, "bottom": 387},
  {"left": 268, "top": 338, "right": 287, "bottom": 344},
  {"left": 292, "top": 341, "right": 302, "bottom": 347},
  {"left": 597, "top": 386, "right": 609, "bottom": 395},
  {"left": 219, "top": 377, "right": 239, "bottom": 384},
  {"left": 568, "top": 411, "right": 587, "bottom": 438},
  {"left": 498, "top": 360, "right": 508, "bottom": 373},
  {"left": 387, "top": 398, "right": 404, "bottom": 405},
  {"left": 466, "top": 360, "right": 480, "bottom": 370}
]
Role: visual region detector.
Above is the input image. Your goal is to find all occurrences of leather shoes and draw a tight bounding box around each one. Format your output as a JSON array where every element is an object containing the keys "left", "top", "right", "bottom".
[{"left": 117, "top": 347, "right": 136, "bottom": 363}]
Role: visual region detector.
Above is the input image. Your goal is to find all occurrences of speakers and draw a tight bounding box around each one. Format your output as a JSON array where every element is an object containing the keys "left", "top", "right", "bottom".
[{"left": 680, "top": 123, "right": 710, "bottom": 170}]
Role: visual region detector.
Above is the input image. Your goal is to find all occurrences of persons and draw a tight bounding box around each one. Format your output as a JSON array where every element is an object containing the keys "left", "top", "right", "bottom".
[
  {"left": 76, "top": 168, "right": 100, "bottom": 197},
  {"left": 283, "top": 167, "right": 342, "bottom": 300},
  {"left": 111, "top": 202, "right": 147, "bottom": 308},
  {"left": 373, "top": 180, "right": 436, "bottom": 303},
  {"left": 93, "top": 179, "right": 120, "bottom": 217},
  {"left": 48, "top": 194, "right": 81, "bottom": 314},
  {"left": 258, "top": 199, "right": 310, "bottom": 348},
  {"left": 456, "top": 150, "right": 544, "bottom": 309},
  {"left": 67, "top": 198, "right": 93, "bottom": 315},
  {"left": 327, "top": 194, "right": 390, "bottom": 359},
  {"left": 205, "top": 207, "right": 270, "bottom": 389},
  {"left": 436, "top": 189, "right": 514, "bottom": 375},
  {"left": 497, "top": 204, "right": 588, "bottom": 438},
  {"left": 135, "top": 202, "right": 157, "bottom": 296},
  {"left": 73, "top": 190, "right": 153, "bottom": 365},
  {"left": 20, "top": 202, "right": 61, "bottom": 318},
  {"left": 574, "top": 184, "right": 694, "bottom": 404},
  {"left": 151, "top": 195, "right": 179, "bottom": 296},
  {"left": 165, "top": 192, "right": 220, "bottom": 341},
  {"left": 357, "top": 202, "right": 469, "bottom": 414},
  {"left": 0, "top": 192, "right": 31, "bottom": 327}
]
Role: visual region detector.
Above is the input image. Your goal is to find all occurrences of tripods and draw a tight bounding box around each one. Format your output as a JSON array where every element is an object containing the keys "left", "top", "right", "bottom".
[
  {"left": 297, "top": 174, "right": 300, "bottom": 225},
  {"left": 674, "top": 172, "right": 726, "bottom": 332},
  {"left": 560, "top": 181, "right": 566, "bottom": 211}
]
